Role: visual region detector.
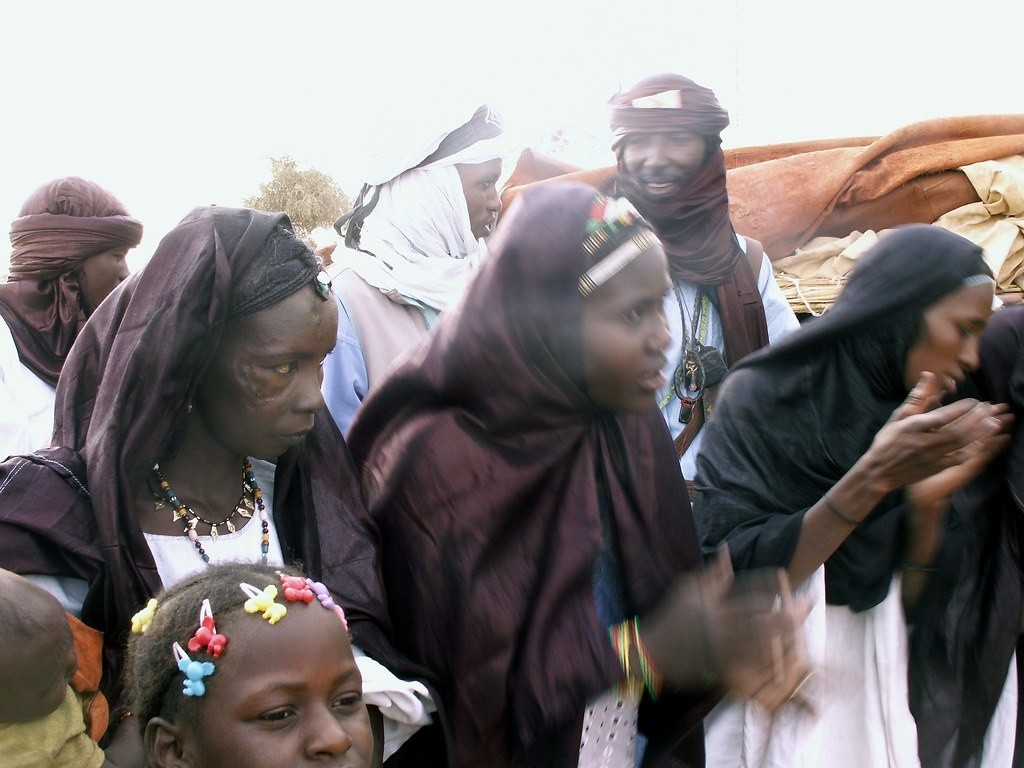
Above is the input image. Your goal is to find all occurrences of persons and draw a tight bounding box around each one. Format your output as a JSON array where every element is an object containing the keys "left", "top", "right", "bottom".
[
  {"left": 906, "top": 306, "right": 1023, "bottom": 768},
  {"left": 0, "top": 568, "right": 147, "bottom": 768},
  {"left": 322, "top": 106, "right": 505, "bottom": 434},
  {"left": 0, "top": 207, "right": 450, "bottom": 768},
  {"left": 691, "top": 225, "right": 1009, "bottom": 768},
  {"left": 123, "top": 561, "right": 375, "bottom": 768},
  {"left": 347, "top": 185, "right": 814, "bottom": 768},
  {"left": 0, "top": 176, "right": 143, "bottom": 461},
  {"left": 607, "top": 78, "right": 801, "bottom": 509}
]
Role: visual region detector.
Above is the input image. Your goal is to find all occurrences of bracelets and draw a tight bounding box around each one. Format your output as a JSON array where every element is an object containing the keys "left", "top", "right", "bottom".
[
  {"left": 822, "top": 495, "right": 860, "bottom": 525},
  {"left": 120, "top": 711, "right": 137, "bottom": 719},
  {"left": 610, "top": 615, "right": 662, "bottom": 698},
  {"left": 904, "top": 562, "right": 939, "bottom": 573}
]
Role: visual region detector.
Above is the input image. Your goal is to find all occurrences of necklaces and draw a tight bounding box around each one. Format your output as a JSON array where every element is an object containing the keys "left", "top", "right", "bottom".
[
  {"left": 659, "top": 280, "right": 709, "bottom": 409},
  {"left": 148, "top": 459, "right": 269, "bottom": 565}
]
